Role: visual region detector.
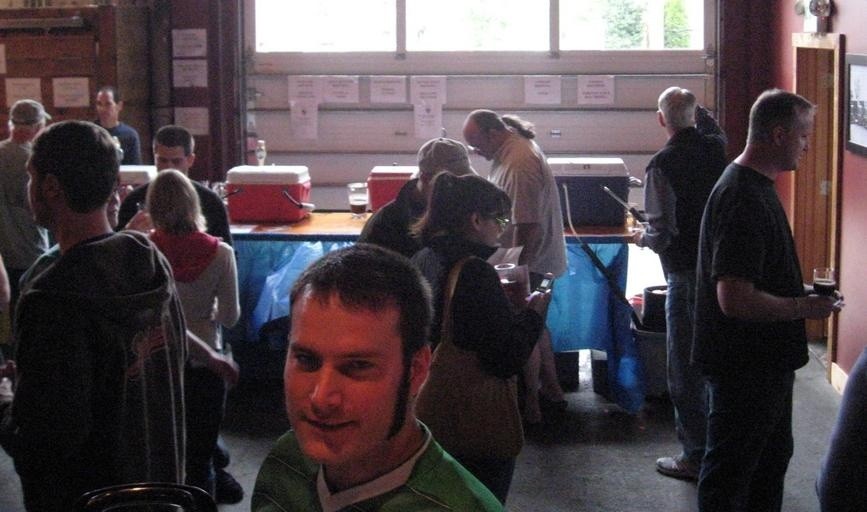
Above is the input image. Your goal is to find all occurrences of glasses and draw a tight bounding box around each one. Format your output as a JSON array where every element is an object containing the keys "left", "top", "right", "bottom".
[{"left": 485, "top": 214, "right": 509, "bottom": 232}]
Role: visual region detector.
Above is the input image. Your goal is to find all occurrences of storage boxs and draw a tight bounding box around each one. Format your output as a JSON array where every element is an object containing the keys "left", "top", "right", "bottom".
[
  {"left": 546, "top": 155, "right": 643, "bottom": 227},
  {"left": 367, "top": 165, "right": 416, "bottom": 212},
  {"left": 224, "top": 163, "right": 312, "bottom": 220}
]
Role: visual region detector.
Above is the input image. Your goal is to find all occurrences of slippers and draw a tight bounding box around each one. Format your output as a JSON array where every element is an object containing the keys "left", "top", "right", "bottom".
[{"left": 539, "top": 390, "right": 567, "bottom": 410}]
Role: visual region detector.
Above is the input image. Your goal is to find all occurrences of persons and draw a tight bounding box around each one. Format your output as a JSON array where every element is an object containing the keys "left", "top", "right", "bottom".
[
  {"left": 352, "top": 136, "right": 478, "bottom": 263},
  {"left": 689, "top": 88, "right": 845, "bottom": 511},
  {"left": 629, "top": 85, "right": 732, "bottom": 482},
  {"left": 0, "top": 86, "right": 246, "bottom": 512},
  {"left": 406, "top": 169, "right": 557, "bottom": 509},
  {"left": 249, "top": 244, "right": 508, "bottom": 511},
  {"left": 458, "top": 109, "right": 567, "bottom": 432},
  {"left": 810, "top": 340, "right": 867, "bottom": 510}
]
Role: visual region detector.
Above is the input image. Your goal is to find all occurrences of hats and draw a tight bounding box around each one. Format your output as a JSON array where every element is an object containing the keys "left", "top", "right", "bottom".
[
  {"left": 418, "top": 137, "right": 480, "bottom": 178},
  {"left": 9, "top": 99, "right": 52, "bottom": 125}
]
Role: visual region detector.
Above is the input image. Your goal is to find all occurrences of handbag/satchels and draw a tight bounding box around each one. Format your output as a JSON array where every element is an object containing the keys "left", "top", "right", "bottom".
[{"left": 412, "top": 340, "right": 525, "bottom": 457}]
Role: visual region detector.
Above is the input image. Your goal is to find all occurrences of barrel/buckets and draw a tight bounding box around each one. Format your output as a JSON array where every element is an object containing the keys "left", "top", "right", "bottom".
[
  {"left": 642, "top": 284, "right": 666, "bottom": 331},
  {"left": 79, "top": 482, "right": 218, "bottom": 512},
  {"left": 634, "top": 330, "right": 668, "bottom": 404}
]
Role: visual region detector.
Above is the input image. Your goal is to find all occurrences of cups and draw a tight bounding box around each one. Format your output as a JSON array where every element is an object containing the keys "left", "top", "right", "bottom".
[
  {"left": 813, "top": 267, "right": 837, "bottom": 297},
  {"left": 347, "top": 182, "right": 369, "bottom": 221},
  {"left": 494, "top": 263, "right": 517, "bottom": 296}
]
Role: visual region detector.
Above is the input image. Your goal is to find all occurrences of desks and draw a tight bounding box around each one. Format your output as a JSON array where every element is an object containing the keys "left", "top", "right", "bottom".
[{"left": 226, "top": 211, "right": 646, "bottom": 404}]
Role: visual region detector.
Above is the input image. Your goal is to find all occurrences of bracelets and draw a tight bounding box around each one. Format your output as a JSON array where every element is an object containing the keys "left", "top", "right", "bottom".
[{"left": 793, "top": 297, "right": 799, "bottom": 318}]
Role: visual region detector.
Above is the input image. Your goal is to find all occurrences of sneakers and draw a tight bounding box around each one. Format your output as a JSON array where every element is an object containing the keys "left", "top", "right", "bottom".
[
  {"left": 656, "top": 451, "right": 701, "bottom": 476},
  {"left": 186, "top": 467, "right": 243, "bottom": 504}
]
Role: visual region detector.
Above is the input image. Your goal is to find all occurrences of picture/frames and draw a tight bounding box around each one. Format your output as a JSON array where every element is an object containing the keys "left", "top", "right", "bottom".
[{"left": 843, "top": 53, "right": 866, "bottom": 158}]
